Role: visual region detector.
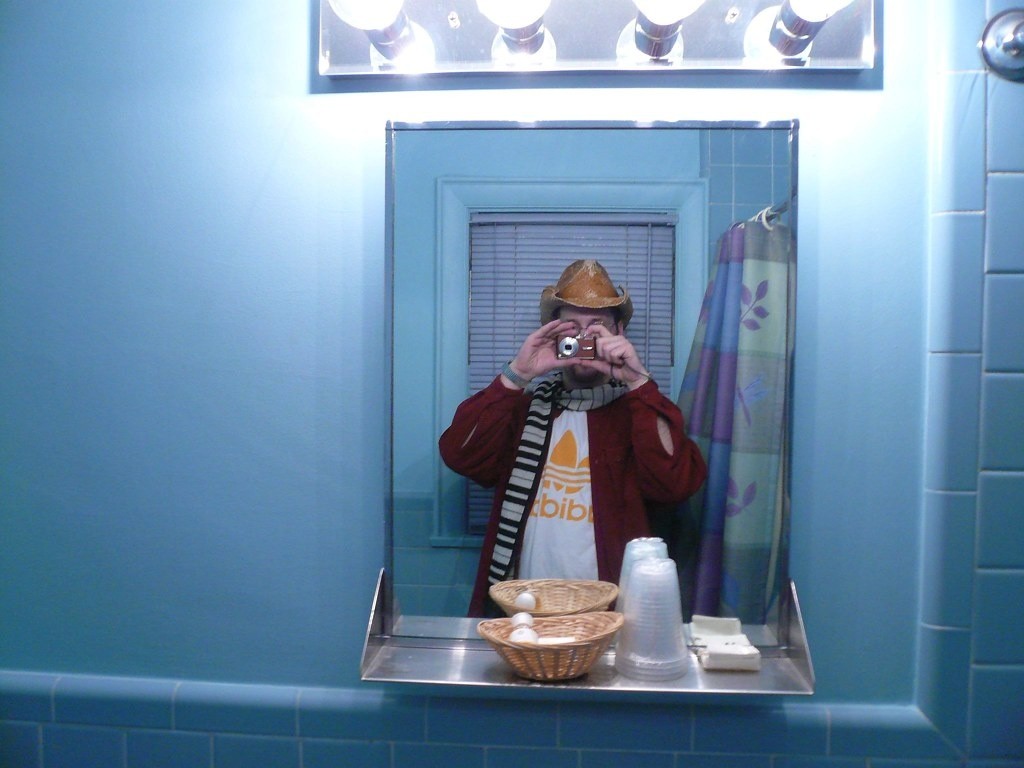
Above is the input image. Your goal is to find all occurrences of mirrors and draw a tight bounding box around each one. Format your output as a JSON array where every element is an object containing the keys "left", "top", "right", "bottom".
[{"left": 384, "top": 119, "right": 800, "bottom": 657}]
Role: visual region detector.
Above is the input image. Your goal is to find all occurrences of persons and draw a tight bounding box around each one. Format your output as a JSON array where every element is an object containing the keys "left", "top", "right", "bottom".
[{"left": 438, "top": 259, "right": 709, "bottom": 619}]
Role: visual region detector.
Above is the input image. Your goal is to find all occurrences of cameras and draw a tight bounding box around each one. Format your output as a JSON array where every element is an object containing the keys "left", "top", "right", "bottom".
[{"left": 556, "top": 334, "right": 595, "bottom": 359}]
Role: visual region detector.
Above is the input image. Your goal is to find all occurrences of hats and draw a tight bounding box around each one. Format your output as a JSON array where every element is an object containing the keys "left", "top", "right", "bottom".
[{"left": 540, "top": 259, "right": 634, "bottom": 326}]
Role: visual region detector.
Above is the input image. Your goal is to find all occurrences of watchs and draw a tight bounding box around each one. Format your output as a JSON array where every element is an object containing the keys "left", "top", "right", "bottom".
[{"left": 500, "top": 359, "right": 531, "bottom": 389}]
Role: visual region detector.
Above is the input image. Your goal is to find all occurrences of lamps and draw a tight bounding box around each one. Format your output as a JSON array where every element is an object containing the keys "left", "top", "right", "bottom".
[{"left": 308, "top": 0, "right": 886, "bottom": 98}]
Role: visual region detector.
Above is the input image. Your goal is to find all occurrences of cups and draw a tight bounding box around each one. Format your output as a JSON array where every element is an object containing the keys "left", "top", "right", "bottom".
[
  {"left": 614, "top": 537, "right": 669, "bottom": 612},
  {"left": 614, "top": 555, "right": 691, "bottom": 683}
]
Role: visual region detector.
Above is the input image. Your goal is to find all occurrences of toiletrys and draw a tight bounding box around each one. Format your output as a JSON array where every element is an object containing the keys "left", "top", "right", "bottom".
[
  {"left": 514, "top": 591, "right": 544, "bottom": 613},
  {"left": 509, "top": 611, "right": 539, "bottom": 647}
]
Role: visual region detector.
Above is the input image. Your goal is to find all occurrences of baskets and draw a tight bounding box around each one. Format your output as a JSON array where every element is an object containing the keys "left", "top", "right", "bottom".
[
  {"left": 489, "top": 578, "right": 619, "bottom": 617},
  {"left": 477, "top": 612, "right": 624, "bottom": 681}
]
